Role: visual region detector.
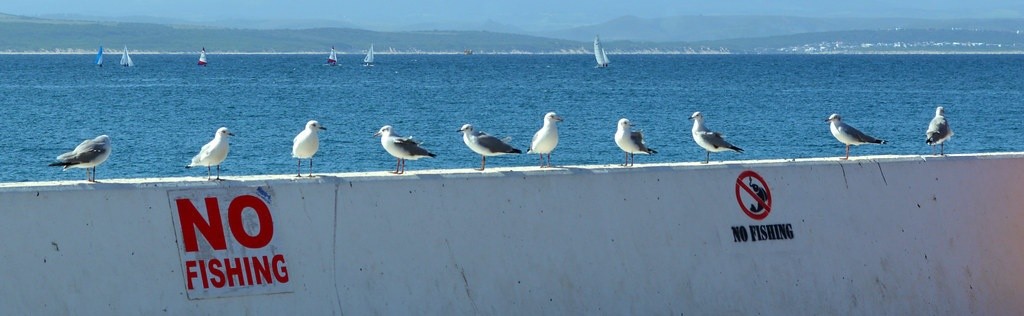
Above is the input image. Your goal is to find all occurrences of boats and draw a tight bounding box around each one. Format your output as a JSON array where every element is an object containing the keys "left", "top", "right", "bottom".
[{"left": 464, "top": 49, "right": 472, "bottom": 55}]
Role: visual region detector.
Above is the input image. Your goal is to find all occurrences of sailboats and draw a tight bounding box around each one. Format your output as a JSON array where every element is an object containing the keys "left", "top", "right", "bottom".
[
  {"left": 93, "top": 46, "right": 103, "bottom": 67},
  {"left": 363, "top": 44, "right": 375, "bottom": 67},
  {"left": 593, "top": 34, "right": 612, "bottom": 68},
  {"left": 197, "top": 47, "right": 208, "bottom": 67},
  {"left": 321, "top": 45, "right": 343, "bottom": 67},
  {"left": 120, "top": 46, "right": 135, "bottom": 67}
]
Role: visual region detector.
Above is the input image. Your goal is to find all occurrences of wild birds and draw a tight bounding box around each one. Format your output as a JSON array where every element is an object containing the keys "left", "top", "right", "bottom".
[
  {"left": 290, "top": 119, "right": 327, "bottom": 178},
  {"left": 183, "top": 125, "right": 236, "bottom": 183},
  {"left": 924, "top": 105, "right": 956, "bottom": 156},
  {"left": 687, "top": 111, "right": 745, "bottom": 165},
  {"left": 614, "top": 117, "right": 659, "bottom": 168},
  {"left": 824, "top": 112, "right": 891, "bottom": 161},
  {"left": 47, "top": 133, "right": 113, "bottom": 184},
  {"left": 373, "top": 124, "right": 438, "bottom": 176},
  {"left": 456, "top": 123, "right": 523, "bottom": 172},
  {"left": 525, "top": 111, "right": 565, "bottom": 169}
]
